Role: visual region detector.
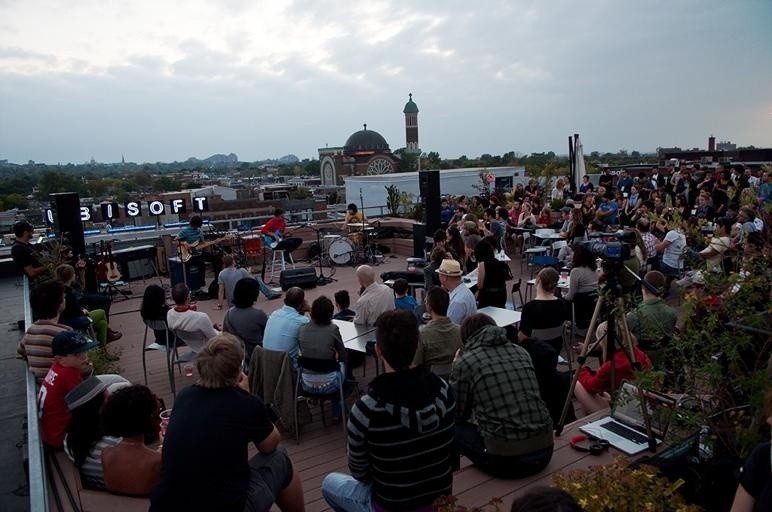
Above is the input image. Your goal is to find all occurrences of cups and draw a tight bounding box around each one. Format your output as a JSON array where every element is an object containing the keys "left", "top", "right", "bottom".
[
  {"left": 189, "top": 302, "right": 197, "bottom": 310},
  {"left": 623, "top": 225, "right": 630, "bottom": 231},
  {"left": 706, "top": 233, "right": 713, "bottom": 242},
  {"left": 560, "top": 271, "right": 568, "bottom": 283},
  {"left": 159, "top": 408, "right": 174, "bottom": 438},
  {"left": 183, "top": 364, "right": 194, "bottom": 376},
  {"left": 407, "top": 263, "right": 416, "bottom": 273}
]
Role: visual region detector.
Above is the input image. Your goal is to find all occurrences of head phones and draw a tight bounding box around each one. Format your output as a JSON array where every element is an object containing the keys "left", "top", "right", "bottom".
[{"left": 569, "top": 434, "right": 609, "bottom": 455}]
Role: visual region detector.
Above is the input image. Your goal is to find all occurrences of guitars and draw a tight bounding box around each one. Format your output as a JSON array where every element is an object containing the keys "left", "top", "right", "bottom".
[
  {"left": 96, "top": 240, "right": 119, "bottom": 281},
  {"left": 261, "top": 223, "right": 306, "bottom": 248},
  {"left": 177, "top": 234, "right": 235, "bottom": 263}
]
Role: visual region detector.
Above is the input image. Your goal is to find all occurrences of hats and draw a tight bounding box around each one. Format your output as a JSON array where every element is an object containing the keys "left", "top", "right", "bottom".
[
  {"left": 64, "top": 375, "right": 107, "bottom": 411},
  {"left": 676, "top": 270, "right": 708, "bottom": 288},
  {"left": 52, "top": 331, "right": 99, "bottom": 354},
  {"left": 434, "top": 259, "right": 463, "bottom": 276}
]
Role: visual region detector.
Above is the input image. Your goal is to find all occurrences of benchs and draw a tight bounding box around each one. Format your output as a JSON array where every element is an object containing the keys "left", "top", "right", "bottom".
[{"left": 332, "top": 387, "right": 717, "bottom": 512}]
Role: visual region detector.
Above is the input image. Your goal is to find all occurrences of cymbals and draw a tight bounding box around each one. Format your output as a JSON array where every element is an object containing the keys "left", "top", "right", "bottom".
[
  {"left": 318, "top": 227, "right": 342, "bottom": 233},
  {"left": 347, "top": 223, "right": 369, "bottom": 227},
  {"left": 360, "top": 227, "right": 374, "bottom": 232}
]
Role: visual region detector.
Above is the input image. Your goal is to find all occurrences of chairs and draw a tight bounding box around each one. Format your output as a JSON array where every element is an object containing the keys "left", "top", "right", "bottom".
[
  {"left": 139, "top": 315, "right": 348, "bottom": 446},
  {"left": 360, "top": 225, "right": 737, "bottom": 388}
]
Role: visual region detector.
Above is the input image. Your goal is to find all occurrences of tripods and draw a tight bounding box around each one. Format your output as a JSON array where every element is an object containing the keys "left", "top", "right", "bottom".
[
  {"left": 555, "top": 297, "right": 656, "bottom": 456},
  {"left": 101, "top": 283, "right": 129, "bottom": 300}
]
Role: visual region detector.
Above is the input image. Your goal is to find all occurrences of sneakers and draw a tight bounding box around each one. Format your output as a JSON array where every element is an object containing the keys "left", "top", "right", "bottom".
[{"left": 106, "top": 327, "right": 122, "bottom": 342}]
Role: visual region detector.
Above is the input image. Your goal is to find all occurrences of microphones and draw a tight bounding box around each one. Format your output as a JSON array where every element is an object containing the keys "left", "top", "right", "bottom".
[{"left": 280, "top": 215, "right": 289, "bottom": 221}]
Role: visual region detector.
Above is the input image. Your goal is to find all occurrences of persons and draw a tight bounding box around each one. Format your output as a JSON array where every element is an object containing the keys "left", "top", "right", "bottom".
[{"left": 9, "top": 162, "right": 771, "bottom": 512}]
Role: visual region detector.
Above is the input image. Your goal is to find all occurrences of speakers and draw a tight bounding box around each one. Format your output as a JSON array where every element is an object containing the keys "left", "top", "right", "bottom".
[
  {"left": 168, "top": 257, "right": 205, "bottom": 291},
  {"left": 279, "top": 268, "right": 317, "bottom": 291},
  {"left": 49, "top": 192, "right": 85, "bottom": 260},
  {"left": 418, "top": 170, "right": 440, "bottom": 226},
  {"left": 494, "top": 176, "right": 513, "bottom": 191},
  {"left": 412, "top": 223, "right": 426, "bottom": 258}
]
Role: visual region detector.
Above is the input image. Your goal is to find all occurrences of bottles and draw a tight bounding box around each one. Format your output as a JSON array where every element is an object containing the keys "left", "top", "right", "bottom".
[{"left": 500, "top": 250, "right": 505, "bottom": 260}]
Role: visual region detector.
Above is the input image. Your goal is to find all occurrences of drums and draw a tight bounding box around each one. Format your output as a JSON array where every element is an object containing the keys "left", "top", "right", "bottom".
[
  {"left": 241, "top": 234, "right": 262, "bottom": 257},
  {"left": 348, "top": 231, "right": 363, "bottom": 247},
  {"left": 323, "top": 235, "right": 342, "bottom": 250},
  {"left": 328, "top": 237, "right": 356, "bottom": 266}
]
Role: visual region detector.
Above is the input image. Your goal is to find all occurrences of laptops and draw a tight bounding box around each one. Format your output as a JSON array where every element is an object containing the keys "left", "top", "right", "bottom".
[{"left": 579, "top": 379, "right": 676, "bottom": 456}]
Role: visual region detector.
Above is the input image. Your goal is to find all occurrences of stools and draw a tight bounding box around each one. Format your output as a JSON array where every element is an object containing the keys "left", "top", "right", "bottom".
[{"left": 269, "top": 249, "right": 298, "bottom": 283}]
